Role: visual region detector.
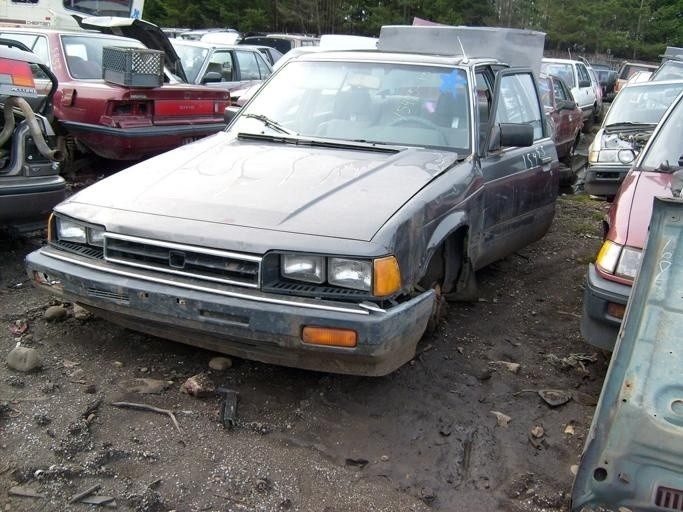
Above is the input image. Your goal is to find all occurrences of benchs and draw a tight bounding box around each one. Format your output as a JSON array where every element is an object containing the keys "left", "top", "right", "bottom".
[{"left": 370, "top": 93, "right": 489, "bottom": 153}]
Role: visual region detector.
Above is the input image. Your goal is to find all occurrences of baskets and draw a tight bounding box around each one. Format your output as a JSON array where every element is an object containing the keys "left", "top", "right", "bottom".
[{"left": 101, "top": 46, "right": 165, "bottom": 88}]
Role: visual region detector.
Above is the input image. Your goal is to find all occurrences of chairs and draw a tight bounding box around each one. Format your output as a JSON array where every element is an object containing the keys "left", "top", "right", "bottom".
[
  {"left": 426, "top": 92, "right": 468, "bottom": 150},
  {"left": 191, "top": 61, "right": 225, "bottom": 82},
  {"left": 316, "top": 87, "right": 377, "bottom": 144}
]
